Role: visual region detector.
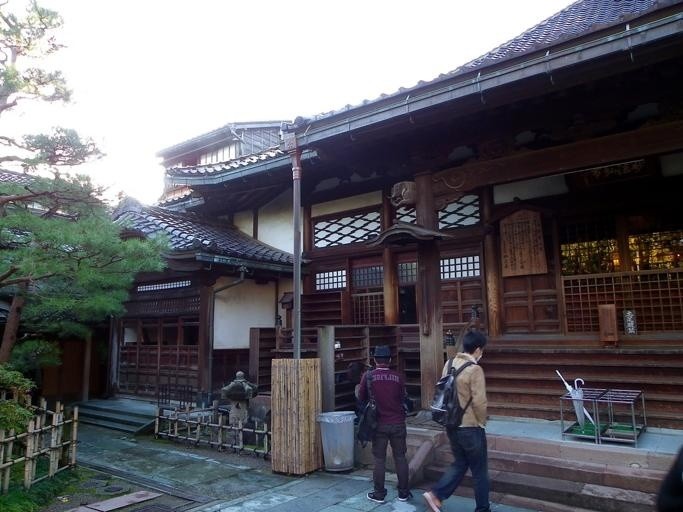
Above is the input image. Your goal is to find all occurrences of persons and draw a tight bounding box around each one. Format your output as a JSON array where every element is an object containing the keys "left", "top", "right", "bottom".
[
  {"left": 354, "top": 345, "right": 414, "bottom": 504},
  {"left": 422, "top": 324, "right": 492, "bottom": 512}
]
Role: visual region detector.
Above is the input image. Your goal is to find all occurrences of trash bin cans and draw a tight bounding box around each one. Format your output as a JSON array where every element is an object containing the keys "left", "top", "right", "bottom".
[{"left": 316, "top": 411, "right": 358, "bottom": 472}]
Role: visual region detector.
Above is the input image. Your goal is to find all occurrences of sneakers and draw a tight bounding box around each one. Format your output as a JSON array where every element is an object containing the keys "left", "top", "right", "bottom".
[
  {"left": 423, "top": 491, "right": 442, "bottom": 512},
  {"left": 398, "top": 496, "right": 408, "bottom": 501},
  {"left": 367, "top": 492, "right": 385, "bottom": 503}
]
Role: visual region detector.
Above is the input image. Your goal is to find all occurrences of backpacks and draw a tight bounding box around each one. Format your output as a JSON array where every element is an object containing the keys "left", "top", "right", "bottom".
[{"left": 429, "top": 357, "right": 476, "bottom": 431}]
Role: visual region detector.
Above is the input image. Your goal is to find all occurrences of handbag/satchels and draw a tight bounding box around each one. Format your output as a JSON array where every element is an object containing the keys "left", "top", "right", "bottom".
[{"left": 355, "top": 401, "right": 379, "bottom": 442}]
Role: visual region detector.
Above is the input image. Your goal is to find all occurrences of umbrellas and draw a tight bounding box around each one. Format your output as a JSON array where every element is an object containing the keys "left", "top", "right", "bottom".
[
  {"left": 569, "top": 378, "right": 585, "bottom": 435},
  {"left": 555, "top": 370, "right": 597, "bottom": 429}
]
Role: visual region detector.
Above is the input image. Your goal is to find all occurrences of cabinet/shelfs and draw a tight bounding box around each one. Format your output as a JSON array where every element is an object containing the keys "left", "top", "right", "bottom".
[
  {"left": 249, "top": 327, "right": 276, "bottom": 418},
  {"left": 398, "top": 324, "right": 421, "bottom": 407},
  {"left": 272, "top": 291, "right": 350, "bottom": 352},
  {"left": 369, "top": 324, "right": 398, "bottom": 371},
  {"left": 316, "top": 324, "right": 369, "bottom": 412},
  {"left": 443, "top": 323, "right": 468, "bottom": 363}
]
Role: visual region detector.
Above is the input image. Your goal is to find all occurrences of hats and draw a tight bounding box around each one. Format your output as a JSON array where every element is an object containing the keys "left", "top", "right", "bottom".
[{"left": 372, "top": 345, "right": 394, "bottom": 357}]
